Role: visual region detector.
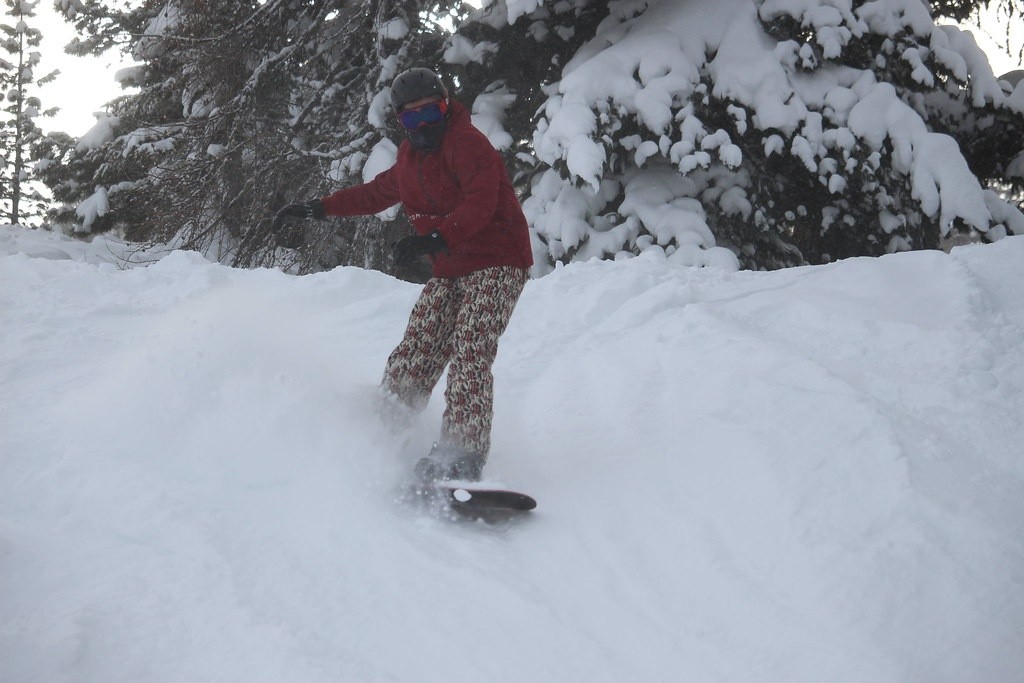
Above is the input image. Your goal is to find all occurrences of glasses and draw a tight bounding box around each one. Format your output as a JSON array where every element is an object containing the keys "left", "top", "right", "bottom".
[{"left": 399, "top": 101, "right": 445, "bottom": 129}]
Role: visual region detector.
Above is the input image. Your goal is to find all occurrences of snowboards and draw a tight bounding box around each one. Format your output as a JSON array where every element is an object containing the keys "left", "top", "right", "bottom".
[{"left": 412, "top": 483, "right": 538, "bottom": 512}]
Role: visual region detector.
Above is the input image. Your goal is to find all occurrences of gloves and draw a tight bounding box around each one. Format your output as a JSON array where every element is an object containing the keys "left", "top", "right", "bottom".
[
  {"left": 273, "top": 199, "right": 325, "bottom": 234},
  {"left": 390, "top": 229, "right": 447, "bottom": 267}
]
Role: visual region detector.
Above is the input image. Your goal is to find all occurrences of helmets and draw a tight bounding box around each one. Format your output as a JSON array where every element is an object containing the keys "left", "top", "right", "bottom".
[{"left": 390, "top": 68, "right": 446, "bottom": 121}]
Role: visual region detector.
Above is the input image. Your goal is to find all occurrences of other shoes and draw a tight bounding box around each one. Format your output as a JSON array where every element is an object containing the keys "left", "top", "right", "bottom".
[{"left": 412, "top": 444, "right": 482, "bottom": 484}]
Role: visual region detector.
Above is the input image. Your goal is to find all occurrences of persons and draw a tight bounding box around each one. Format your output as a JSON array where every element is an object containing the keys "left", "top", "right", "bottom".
[{"left": 272, "top": 68, "right": 534, "bottom": 478}]
